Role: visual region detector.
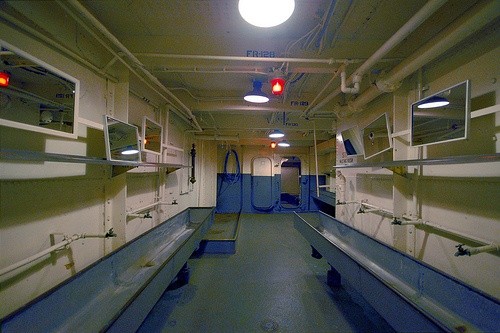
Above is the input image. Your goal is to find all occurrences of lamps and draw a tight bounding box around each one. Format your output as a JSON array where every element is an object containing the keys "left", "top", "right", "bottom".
[
  {"left": 243, "top": 80, "right": 269, "bottom": 104},
  {"left": 278, "top": 139, "right": 291, "bottom": 148},
  {"left": 270, "top": 138, "right": 277, "bottom": 149},
  {"left": 269, "top": 72, "right": 287, "bottom": 97},
  {"left": 417, "top": 95, "right": 451, "bottom": 109},
  {"left": 268, "top": 129, "right": 286, "bottom": 138}
]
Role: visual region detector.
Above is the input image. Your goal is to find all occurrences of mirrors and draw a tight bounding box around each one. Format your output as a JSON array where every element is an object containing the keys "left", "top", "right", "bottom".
[
  {"left": 141, "top": 115, "right": 163, "bottom": 156},
  {"left": 341, "top": 126, "right": 364, "bottom": 157},
  {"left": 362, "top": 111, "right": 392, "bottom": 159},
  {"left": 409, "top": 79, "right": 471, "bottom": 148},
  {"left": 103, "top": 115, "right": 143, "bottom": 165},
  {"left": 0, "top": 38, "right": 80, "bottom": 140}
]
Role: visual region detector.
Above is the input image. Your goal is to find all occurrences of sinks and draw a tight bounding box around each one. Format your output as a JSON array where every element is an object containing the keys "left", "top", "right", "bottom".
[
  {"left": 312, "top": 190, "right": 336, "bottom": 207},
  {"left": 0, "top": 206, "right": 217, "bottom": 333},
  {"left": 292, "top": 210, "right": 500, "bottom": 333}
]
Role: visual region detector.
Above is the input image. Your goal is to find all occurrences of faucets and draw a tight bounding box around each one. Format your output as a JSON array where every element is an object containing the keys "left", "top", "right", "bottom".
[
  {"left": 106, "top": 226, "right": 119, "bottom": 240},
  {"left": 390, "top": 216, "right": 401, "bottom": 227},
  {"left": 356, "top": 207, "right": 364, "bottom": 215},
  {"left": 454, "top": 242, "right": 470, "bottom": 258},
  {"left": 143, "top": 210, "right": 152, "bottom": 220},
  {"left": 172, "top": 199, "right": 178, "bottom": 205},
  {"left": 335, "top": 200, "right": 344, "bottom": 207}
]
